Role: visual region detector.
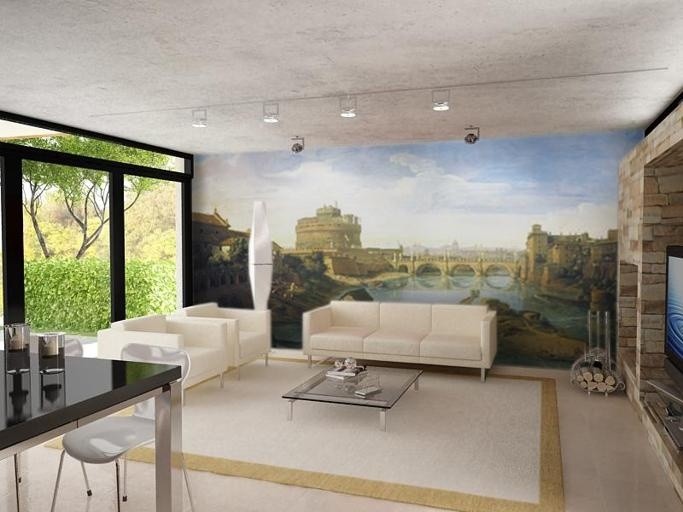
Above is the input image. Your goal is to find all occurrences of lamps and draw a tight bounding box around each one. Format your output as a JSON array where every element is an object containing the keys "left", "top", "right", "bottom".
[{"left": 189, "top": 87, "right": 479, "bottom": 153}]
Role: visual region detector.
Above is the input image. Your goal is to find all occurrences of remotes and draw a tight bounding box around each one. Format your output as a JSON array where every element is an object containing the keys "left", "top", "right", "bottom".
[{"left": 356, "top": 386, "right": 381, "bottom": 397}]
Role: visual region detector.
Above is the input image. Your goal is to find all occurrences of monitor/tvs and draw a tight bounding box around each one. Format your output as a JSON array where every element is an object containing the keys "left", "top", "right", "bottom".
[{"left": 663, "top": 245, "right": 683, "bottom": 373}]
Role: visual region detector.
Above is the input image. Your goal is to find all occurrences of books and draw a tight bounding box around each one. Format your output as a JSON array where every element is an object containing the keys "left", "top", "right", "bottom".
[
  {"left": 355, "top": 386, "right": 382, "bottom": 396},
  {"left": 325, "top": 372, "right": 357, "bottom": 379},
  {"left": 326, "top": 362, "right": 365, "bottom": 376}
]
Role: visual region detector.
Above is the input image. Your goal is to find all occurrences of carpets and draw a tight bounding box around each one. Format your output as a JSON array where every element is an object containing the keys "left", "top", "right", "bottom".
[{"left": 44, "top": 357, "right": 570, "bottom": 511}]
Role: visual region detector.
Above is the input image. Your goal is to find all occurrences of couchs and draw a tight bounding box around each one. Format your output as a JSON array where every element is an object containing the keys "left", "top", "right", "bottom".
[
  {"left": 170, "top": 303, "right": 270, "bottom": 382},
  {"left": 90, "top": 311, "right": 234, "bottom": 406},
  {"left": 300, "top": 299, "right": 500, "bottom": 382}
]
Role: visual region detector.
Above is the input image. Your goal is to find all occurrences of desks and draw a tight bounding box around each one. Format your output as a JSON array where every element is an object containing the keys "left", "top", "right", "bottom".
[{"left": 0, "top": 349, "right": 181, "bottom": 511}]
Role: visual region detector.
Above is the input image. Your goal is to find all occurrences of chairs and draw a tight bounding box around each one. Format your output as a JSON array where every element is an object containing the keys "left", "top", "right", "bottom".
[
  {"left": 50, "top": 342, "right": 195, "bottom": 512},
  {"left": 53, "top": 336, "right": 95, "bottom": 498}
]
